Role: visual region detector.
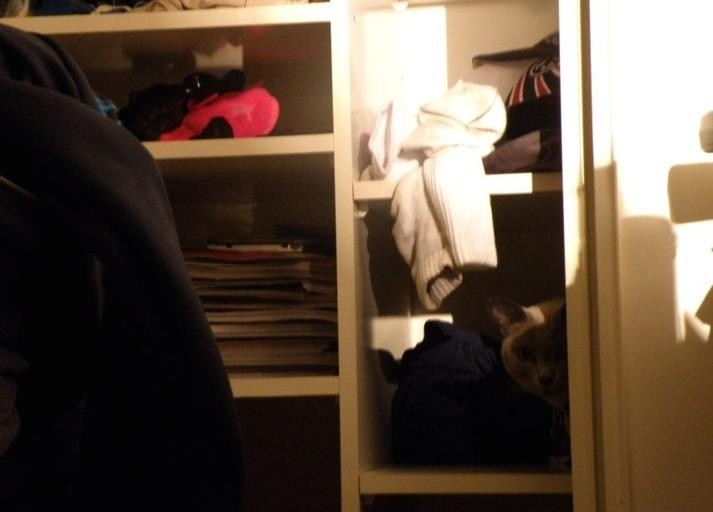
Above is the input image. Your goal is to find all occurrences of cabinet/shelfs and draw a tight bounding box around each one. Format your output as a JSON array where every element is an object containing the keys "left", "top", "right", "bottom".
[{"left": 0, "top": 0, "right": 604, "bottom": 512}]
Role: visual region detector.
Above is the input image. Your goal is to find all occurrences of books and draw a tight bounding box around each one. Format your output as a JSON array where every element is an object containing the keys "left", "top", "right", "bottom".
[{"left": 177, "top": 247, "right": 340, "bottom": 374}]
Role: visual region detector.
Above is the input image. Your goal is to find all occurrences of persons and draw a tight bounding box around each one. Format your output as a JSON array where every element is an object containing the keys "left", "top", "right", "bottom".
[{"left": 1, "top": 23, "right": 247, "bottom": 512}]
